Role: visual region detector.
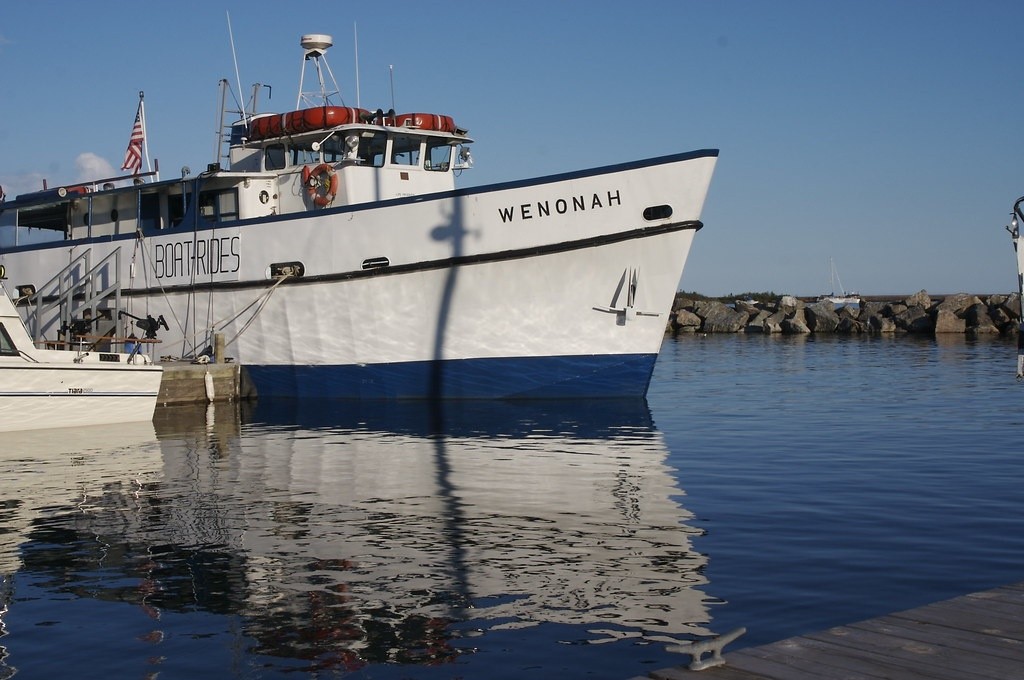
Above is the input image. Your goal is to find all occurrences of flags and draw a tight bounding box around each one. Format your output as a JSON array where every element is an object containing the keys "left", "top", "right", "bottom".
[{"left": 119, "top": 100, "right": 144, "bottom": 176}]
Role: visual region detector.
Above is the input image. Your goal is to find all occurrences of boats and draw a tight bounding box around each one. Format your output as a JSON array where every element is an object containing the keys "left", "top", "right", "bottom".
[
  {"left": 0, "top": 10, "right": 721, "bottom": 418},
  {"left": 0, "top": 283, "right": 163, "bottom": 434}
]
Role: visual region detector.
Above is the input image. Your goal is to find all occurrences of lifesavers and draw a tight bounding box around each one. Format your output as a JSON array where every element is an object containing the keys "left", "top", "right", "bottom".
[{"left": 306, "top": 163, "right": 338, "bottom": 206}]
